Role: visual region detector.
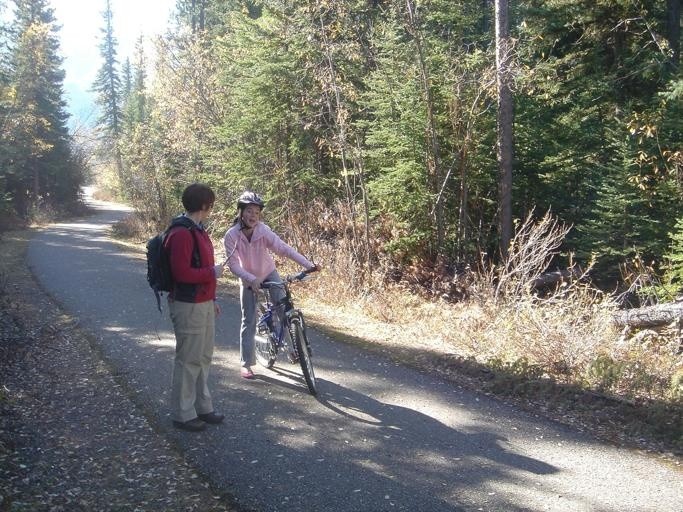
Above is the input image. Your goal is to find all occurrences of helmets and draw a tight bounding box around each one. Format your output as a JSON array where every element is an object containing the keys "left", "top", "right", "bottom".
[{"left": 237, "top": 190, "right": 265, "bottom": 209}]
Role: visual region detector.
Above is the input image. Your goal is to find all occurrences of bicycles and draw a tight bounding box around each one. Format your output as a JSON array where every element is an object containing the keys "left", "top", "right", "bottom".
[{"left": 247, "top": 267, "right": 317, "bottom": 395}]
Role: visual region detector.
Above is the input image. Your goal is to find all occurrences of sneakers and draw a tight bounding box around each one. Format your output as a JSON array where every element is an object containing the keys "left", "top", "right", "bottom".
[
  {"left": 173, "top": 411, "right": 225, "bottom": 431},
  {"left": 240, "top": 367, "right": 255, "bottom": 378}
]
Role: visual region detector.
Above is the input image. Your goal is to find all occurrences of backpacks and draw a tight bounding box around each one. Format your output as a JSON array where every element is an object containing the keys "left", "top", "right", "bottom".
[{"left": 146, "top": 219, "right": 201, "bottom": 296}]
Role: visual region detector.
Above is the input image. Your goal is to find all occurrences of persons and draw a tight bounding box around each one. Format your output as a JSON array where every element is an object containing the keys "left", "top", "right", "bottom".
[
  {"left": 225, "top": 191, "right": 321, "bottom": 379},
  {"left": 165, "top": 184, "right": 224, "bottom": 431}
]
